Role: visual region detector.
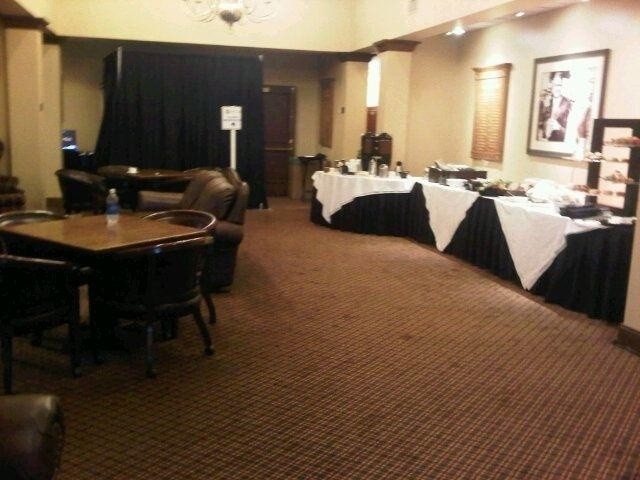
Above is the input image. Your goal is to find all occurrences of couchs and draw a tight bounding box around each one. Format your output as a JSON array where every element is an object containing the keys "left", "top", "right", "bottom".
[
  {"left": 0, "top": 391, "right": 64, "bottom": 480},
  {"left": 137, "top": 167, "right": 250, "bottom": 292}
]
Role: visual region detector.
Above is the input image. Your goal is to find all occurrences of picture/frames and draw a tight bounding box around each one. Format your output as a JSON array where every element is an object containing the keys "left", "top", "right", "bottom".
[{"left": 527, "top": 48, "right": 611, "bottom": 162}]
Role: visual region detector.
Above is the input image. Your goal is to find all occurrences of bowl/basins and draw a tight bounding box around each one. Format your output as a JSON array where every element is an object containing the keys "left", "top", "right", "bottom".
[{"left": 398, "top": 170, "right": 410, "bottom": 179}]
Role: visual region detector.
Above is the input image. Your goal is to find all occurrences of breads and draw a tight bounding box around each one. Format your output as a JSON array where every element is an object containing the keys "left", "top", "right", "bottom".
[{"left": 571, "top": 136, "right": 639, "bottom": 196}]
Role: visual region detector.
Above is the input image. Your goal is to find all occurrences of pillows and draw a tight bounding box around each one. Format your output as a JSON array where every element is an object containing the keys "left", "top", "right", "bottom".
[
  {"left": 193, "top": 177, "right": 237, "bottom": 220},
  {"left": 178, "top": 170, "right": 225, "bottom": 211}
]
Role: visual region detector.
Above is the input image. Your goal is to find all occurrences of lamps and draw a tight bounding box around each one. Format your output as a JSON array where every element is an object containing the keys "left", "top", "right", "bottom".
[
  {"left": 184, "top": 0, "right": 272, "bottom": 29},
  {"left": 453, "top": 20, "right": 466, "bottom": 35}
]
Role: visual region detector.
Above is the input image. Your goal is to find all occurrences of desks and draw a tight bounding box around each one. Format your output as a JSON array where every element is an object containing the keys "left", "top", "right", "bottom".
[
  {"left": 310, "top": 169, "right": 634, "bottom": 324},
  {"left": 109, "top": 168, "right": 185, "bottom": 177}
]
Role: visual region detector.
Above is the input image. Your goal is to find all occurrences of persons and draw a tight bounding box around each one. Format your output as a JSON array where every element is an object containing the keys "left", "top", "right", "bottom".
[{"left": 537, "top": 71, "right": 571, "bottom": 142}]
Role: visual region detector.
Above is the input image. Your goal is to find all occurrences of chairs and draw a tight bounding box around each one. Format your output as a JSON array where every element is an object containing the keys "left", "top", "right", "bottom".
[
  {"left": 55, "top": 168, "right": 106, "bottom": 213},
  {"left": 144, "top": 179, "right": 179, "bottom": 192},
  {"left": 0, "top": 176, "right": 27, "bottom": 212}
]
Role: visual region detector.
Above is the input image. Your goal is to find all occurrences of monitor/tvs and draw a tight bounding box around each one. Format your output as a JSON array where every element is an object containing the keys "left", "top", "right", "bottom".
[{"left": 61, "top": 130, "right": 78, "bottom": 151}]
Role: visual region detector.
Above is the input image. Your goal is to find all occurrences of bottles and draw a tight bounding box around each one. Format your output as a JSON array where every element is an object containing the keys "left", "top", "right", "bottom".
[
  {"left": 368, "top": 159, "right": 377, "bottom": 177},
  {"left": 105, "top": 188, "right": 120, "bottom": 224},
  {"left": 395, "top": 161, "right": 403, "bottom": 176},
  {"left": 378, "top": 163, "right": 389, "bottom": 177}
]
